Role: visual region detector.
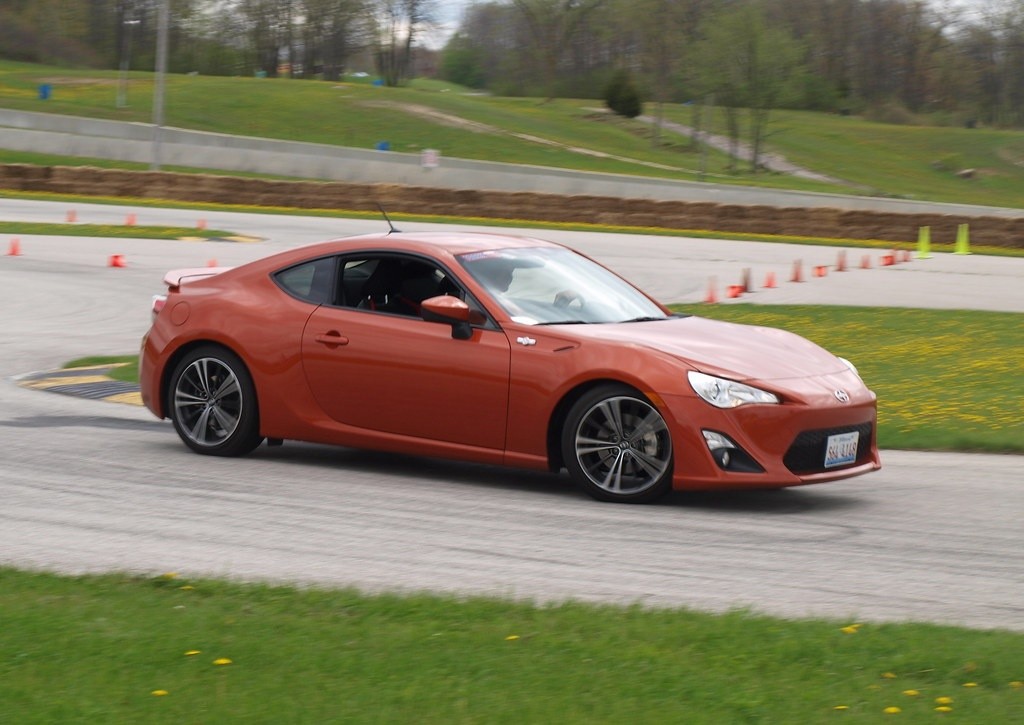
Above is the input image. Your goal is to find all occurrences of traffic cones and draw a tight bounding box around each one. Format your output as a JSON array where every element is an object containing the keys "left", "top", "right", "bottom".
[
  {"left": 196, "top": 217, "right": 207, "bottom": 229},
  {"left": 706, "top": 246, "right": 912, "bottom": 301},
  {"left": 111, "top": 254, "right": 125, "bottom": 267},
  {"left": 65, "top": 210, "right": 75, "bottom": 221},
  {"left": 123, "top": 213, "right": 136, "bottom": 226},
  {"left": 952, "top": 224, "right": 972, "bottom": 255},
  {"left": 6, "top": 237, "right": 22, "bottom": 254},
  {"left": 917, "top": 227, "right": 934, "bottom": 258}
]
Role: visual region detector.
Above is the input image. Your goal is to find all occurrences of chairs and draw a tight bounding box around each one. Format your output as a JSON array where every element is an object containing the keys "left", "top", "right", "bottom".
[{"left": 356, "top": 255, "right": 459, "bottom": 319}]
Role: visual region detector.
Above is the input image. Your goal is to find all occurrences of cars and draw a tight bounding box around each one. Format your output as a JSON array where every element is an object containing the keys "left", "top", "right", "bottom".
[{"left": 137, "top": 230, "right": 882, "bottom": 504}]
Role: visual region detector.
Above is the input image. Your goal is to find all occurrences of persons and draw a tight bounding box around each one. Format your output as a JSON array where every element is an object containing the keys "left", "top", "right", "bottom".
[{"left": 464, "top": 268, "right": 514, "bottom": 329}]
